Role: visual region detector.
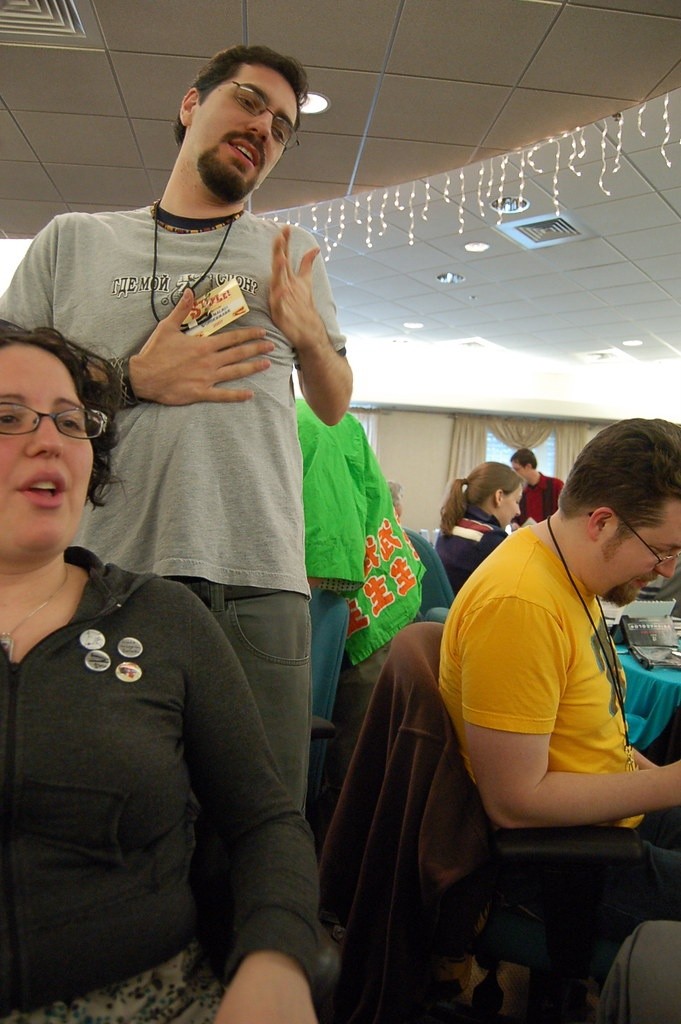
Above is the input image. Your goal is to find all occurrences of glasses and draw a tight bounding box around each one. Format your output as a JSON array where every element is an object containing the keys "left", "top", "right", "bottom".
[
  {"left": 588, "top": 506, "right": 681, "bottom": 566},
  {"left": 222, "top": 80, "right": 300, "bottom": 151},
  {"left": 0, "top": 402, "right": 108, "bottom": 439}
]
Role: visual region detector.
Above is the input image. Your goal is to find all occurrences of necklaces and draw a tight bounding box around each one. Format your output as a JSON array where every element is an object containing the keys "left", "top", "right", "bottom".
[
  {"left": 1, "top": 564, "right": 69, "bottom": 658},
  {"left": 547, "top": 515, "right": 640, "bottom": 772}
]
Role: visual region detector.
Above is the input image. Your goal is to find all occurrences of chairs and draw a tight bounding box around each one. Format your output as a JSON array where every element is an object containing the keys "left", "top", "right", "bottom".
[
  {"left": 403, "top": 527, "right": 456, "bottom": 616},
  {"left": 386, "top": 622, "right": 647, "bottom": 1024}
]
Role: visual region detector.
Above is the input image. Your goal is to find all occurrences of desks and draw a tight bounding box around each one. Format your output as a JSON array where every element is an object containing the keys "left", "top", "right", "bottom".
[{"left": 616, "top": 638, "right": 681, "bottom": 751}]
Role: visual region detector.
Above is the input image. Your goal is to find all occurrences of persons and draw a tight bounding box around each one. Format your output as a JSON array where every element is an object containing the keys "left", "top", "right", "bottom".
[
  {"left": 509, "top": 445, "right": 565, "bottom": 532},
  {"left": 294, "top": 397, "right": 425, "bottom": 807},
  {"left": 438, "top": 420, "right": 681, "bottom": 936},
  {"left": 1, "top": 319, "right": 340, "bottom": 1024},
  {"left": 433, "top": 461, "right": 524, "bottom": 597},
  {"left": 384, "top": 481, "right": 404, "bottom": 522},
  {"left": 599, "top": 921, "right": 681, "bottom": 1024},
  {"left": 639, "top": 554, "right": 680, "bottom": 617},
  {"left": 1, "top": 43, "right": 354, "bottom": 822}
]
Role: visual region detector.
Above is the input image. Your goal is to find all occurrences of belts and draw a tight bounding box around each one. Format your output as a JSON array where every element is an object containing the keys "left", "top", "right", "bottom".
[{"left": 187, "top": 582, "right": 280, "bottom": 602}]
